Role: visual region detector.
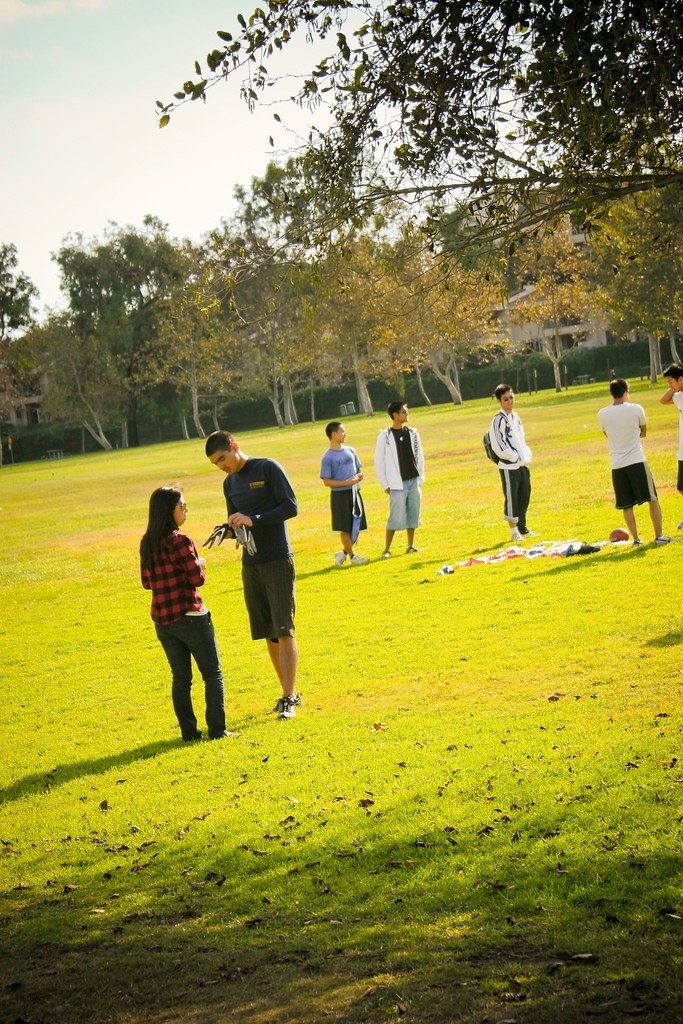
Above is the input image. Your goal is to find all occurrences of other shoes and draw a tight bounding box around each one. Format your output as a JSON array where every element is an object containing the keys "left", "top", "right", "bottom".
[
  {"left": 381, "top": 551, "right": 390, "bottom": 558},
  {"left": 632, "top": 539, "right": 644, "bottom": 546},
  {"left": 678, "top": 522, "right": 683, "bottom": 529},
  {"left": 406, "top": 547, "right": 417, "bottom": 554},
  {"left": 222, "top": 730, "right": 242, "bottom": 737},
  {"left": 655, "top": 535, "right": 670, "bottom": 545},
  {"left": 512, "top": 534, "right": 525, "bottom": 542},
  {"left": 523, "top": 533, "right": 539, "bottom": 538}
]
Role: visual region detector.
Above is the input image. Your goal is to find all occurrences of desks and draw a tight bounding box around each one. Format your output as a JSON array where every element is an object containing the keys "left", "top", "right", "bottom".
[
  {"left": 577, "top": 374, "right": 591, "bottom": 385},
  {"left": 46, "top": 449, "right": 64, "bottom": 459}
]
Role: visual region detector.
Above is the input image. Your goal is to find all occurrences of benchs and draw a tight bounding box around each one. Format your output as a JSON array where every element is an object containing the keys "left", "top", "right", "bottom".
[
  {"left": 572, "top": 380, "right": 577, "bottom": 386},
  {"left": 589, "top": 377, "right": 596, "bottom": 384}
]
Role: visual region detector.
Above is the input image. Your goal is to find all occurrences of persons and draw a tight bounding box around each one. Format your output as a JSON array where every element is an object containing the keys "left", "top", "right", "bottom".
[
  {"left": 660, "top": 362, "right": 683, "bottom": 529},
  {"left": 488, "top": 384, "right": 539, "bottom": 541},
  {"left": 205, "top": 431, "right": 300, "bottom": 722},
  {"left": 373, "top": 401, "right": 425, "bottom": 558},
  {"left": 140, "top": 485, "right": 230, "bottom": 742},
  {"left": 596, "top": 379, "right": 670, "bottom": 544},
  {"left": 320, "top": 423, "right": 369, "bottom": 566}
]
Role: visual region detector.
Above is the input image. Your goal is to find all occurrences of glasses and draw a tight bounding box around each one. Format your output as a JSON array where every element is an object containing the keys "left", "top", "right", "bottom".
[
  {"left": 400, "top": 410, "right": 408, "bottom": 413},
  {"left": 503, "top": 396, "right": 513, "bottom": 401},
  {"left": 176, "top": 503, "right": 186, "bottom": 510}
]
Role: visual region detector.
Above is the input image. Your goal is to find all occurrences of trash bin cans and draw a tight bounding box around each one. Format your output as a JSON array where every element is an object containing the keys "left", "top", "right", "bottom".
[
  {"left": 339, "top": 404, "right": 348, "bottom": 416},
  {"left": 347, "top": 402, "right": 355, "bottom": 414}
]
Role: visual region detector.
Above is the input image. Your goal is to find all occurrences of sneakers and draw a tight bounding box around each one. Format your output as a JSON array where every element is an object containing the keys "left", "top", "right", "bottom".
[
  {"left": 267, "top": 692, "right": 302, "bottom": 718},
  {"left": 350, "top": 555, "right": 370, "bottom": 565},
  {"left": 335, "top": 551, "right": 347, "bottom": 566}
]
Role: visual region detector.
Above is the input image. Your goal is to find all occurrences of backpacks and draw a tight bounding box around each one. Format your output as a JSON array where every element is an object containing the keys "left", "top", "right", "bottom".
[{"left": 483, "top": 412, "right": 512, "bottom": 465}]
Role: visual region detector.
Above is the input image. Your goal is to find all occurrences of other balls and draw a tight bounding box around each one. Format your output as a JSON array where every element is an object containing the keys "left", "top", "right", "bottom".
[{"left": 609, "top": 527, "right": 629, "bottom": 542}]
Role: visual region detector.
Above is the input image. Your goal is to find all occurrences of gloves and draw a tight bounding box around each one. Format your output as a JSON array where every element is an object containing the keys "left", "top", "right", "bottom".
[{"left": 202, "top": 524, "right": 257, "bottom": 556}]
